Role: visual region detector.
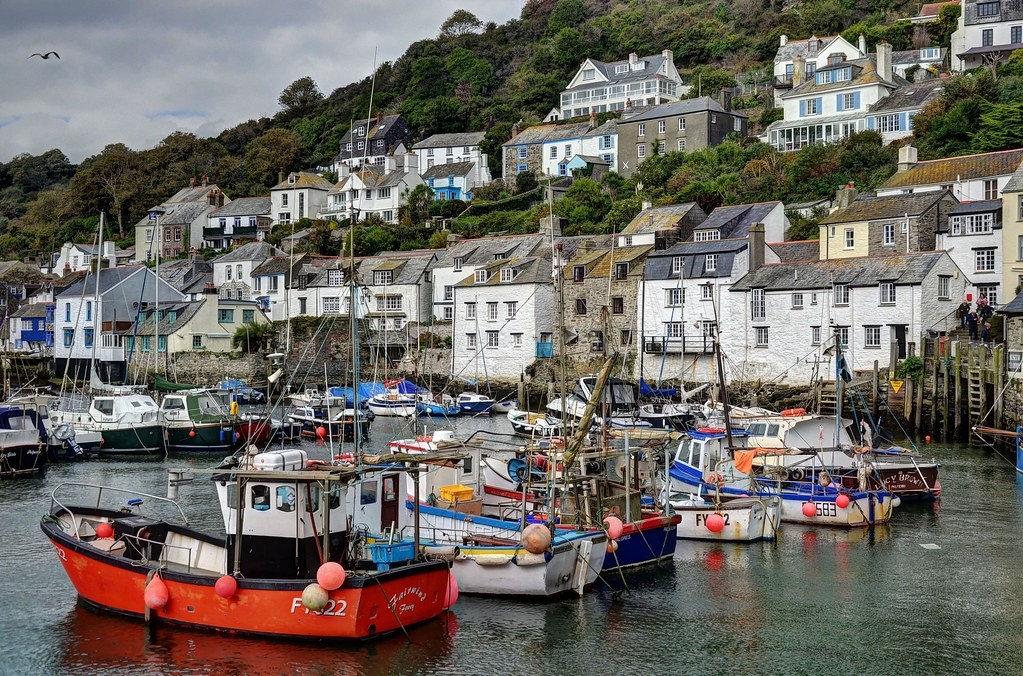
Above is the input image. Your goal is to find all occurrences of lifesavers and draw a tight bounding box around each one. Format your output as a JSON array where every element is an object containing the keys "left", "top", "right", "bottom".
[
  {"left": 698, "top": 428, "right": 725, "bottom": 434},
  {"left": 708, "top": 473, "right": 723, "bottom": 486},
  {"left": 231, "top": 402, "right": 238, "bottom": 414},
  {"left": 417, "top": 435, "right": 433, "bottom": 442},
  {"left": 334, "top": 452, "right": 359, "bottom": 462},
  {"left": 781, "top": 408, "right": 804, "bottom": 417},
  {"left": 792, "top": 468, "right": 805, "bottom": 480}
]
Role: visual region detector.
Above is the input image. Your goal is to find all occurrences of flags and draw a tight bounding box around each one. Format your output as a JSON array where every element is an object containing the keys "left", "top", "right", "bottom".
[{"left": 839, "top": 355, "right": 852, "bottom": 383}]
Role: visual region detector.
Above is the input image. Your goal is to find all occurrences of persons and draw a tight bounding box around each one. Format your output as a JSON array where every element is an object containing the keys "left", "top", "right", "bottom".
[
  {"left": 976, "top": 293, "right": 994, "bottom": 325},
  {"left": 958, "top": 300, "right": 970, "bottom": 330},
  {"left": 980, "top": 323, "right": 991, "bottom": 348},
  {"left": 967, "top": 310, "right": 979, "bottom": 342}
]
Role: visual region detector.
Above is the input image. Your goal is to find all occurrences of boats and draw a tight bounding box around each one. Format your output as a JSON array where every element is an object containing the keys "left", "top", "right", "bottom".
[{"left": 1, "top": 198, "right": 942, "bottom": 599}]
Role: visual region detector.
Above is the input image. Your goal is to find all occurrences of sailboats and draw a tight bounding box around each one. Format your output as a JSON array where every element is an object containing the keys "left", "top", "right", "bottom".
[{"left": 32, "top": 128, "right": 463, "bottom": 636}]
